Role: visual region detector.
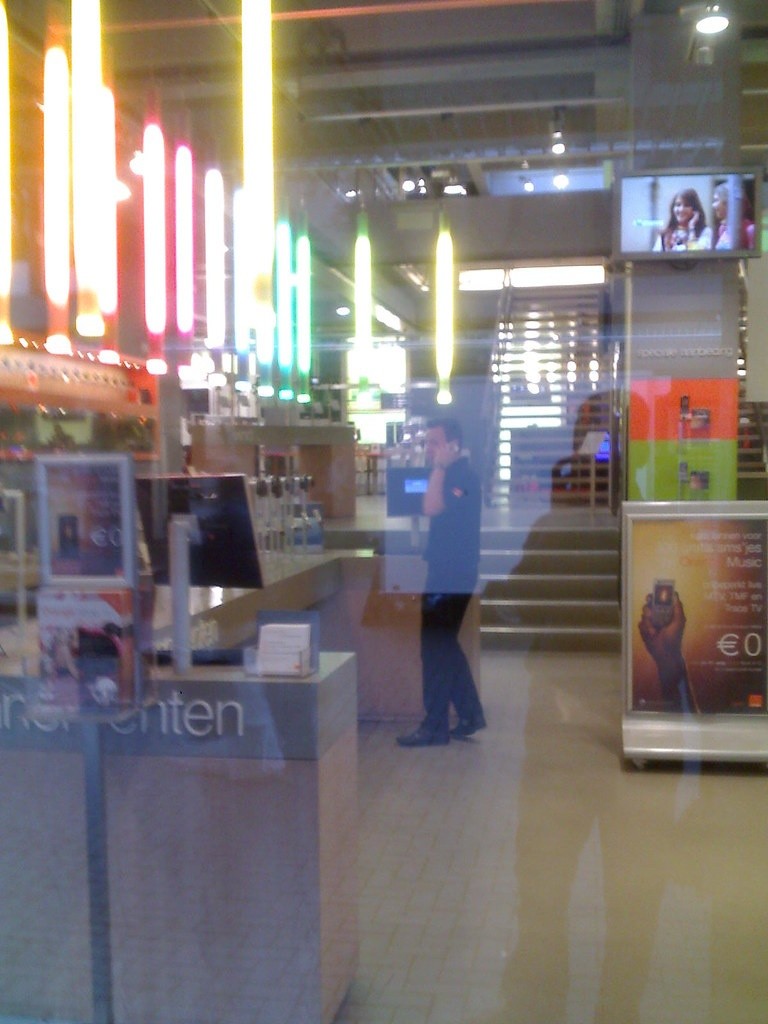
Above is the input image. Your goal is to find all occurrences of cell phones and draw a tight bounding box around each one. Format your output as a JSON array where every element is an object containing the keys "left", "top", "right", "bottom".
[{"left": 650, "top": 578, "right": 677, "bottom": 627}]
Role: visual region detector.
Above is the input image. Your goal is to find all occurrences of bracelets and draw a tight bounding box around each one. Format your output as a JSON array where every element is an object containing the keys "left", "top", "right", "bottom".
[{"left": 688, "top": 228, "right": 697, "bottom": 231}]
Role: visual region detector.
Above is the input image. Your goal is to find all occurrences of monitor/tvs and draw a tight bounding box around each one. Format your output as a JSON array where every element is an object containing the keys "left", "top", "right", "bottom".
[
  {"left": 609, "top": 165, "right": 764, "bottom": 260},
  {"left": 132, "top": 471, "right": 264, "bottom": 590}
]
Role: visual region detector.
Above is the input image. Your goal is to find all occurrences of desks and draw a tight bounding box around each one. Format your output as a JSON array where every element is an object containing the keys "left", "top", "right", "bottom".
[
  {"left": 186, "top": 420, "right": 358, "bottom": 520},
  {"left": 365, "top": 450, "right": 416, "bottom": 495}
]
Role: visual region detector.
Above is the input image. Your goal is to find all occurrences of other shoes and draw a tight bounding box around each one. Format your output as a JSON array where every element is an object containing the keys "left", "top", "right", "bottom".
[
  {"left": 396, "top": 726, "right": 450, "bottom": 746},
  {"left": 450, "top": 711, "right": 486, "bottom": 735}
]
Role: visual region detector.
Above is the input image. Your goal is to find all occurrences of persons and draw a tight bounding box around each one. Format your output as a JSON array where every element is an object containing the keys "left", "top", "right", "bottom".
[
  {"left": 652, "top": 188, "right": 714, "bottom": 252},
  {"left": 396, "top": 417, "right": 486, "bottom": 747},
  {"left": 637, "top": 591, "right": 700, "bottom": 713},
  {"left": 503, "top": 390, "right": 713, "bottom": 1024},
  {"left": 712, "top": 181, "right": 756, "bottom": 250}
]
